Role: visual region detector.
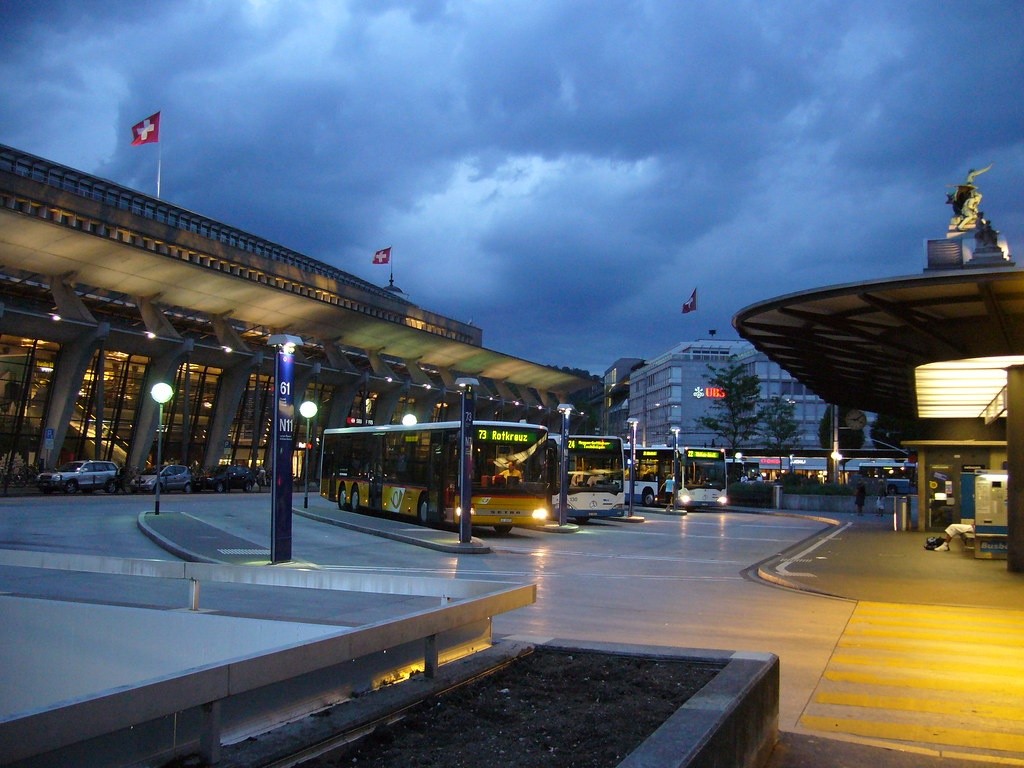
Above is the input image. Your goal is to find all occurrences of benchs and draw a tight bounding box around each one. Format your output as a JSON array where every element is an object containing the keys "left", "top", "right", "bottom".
[{"left": 960, "top": 518, "right": 975, "bottom": 557}]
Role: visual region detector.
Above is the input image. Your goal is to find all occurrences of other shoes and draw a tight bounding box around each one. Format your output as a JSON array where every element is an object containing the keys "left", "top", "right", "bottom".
[{"left": 934, "top": 545, "right": 949, "bottom": 551}]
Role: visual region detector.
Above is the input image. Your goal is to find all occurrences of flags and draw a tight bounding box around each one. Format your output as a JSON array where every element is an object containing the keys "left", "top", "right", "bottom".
[
  {"left": 132, "top": 112, "right": 160, "bottom": 146},
  {"left": 372, "top": 247, "right": 391, "bottom": 264},
  {"left": 682, "top": 288, "right": 696, "bottom": 314}
]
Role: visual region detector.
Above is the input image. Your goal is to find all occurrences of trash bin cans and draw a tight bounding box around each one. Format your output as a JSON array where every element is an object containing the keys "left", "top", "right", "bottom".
[
  {"left": 894, "top": 495, "right": 911, "bottom": 531},
  {"left": 773, "top": 485, "right": 783, "bottom": 509}
]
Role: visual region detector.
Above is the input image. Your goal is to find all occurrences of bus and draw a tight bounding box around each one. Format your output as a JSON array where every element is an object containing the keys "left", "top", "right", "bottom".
[
  {"left": 494, "top": 434, "right": 626, "bottom": 525},
  {"left": 610, "top": 443, "right": 727, "bottom": 511},
  {"left": 857, "top": 462, "right": 918, "bottom": 495},
  {"left": 319, "top": 421, "right": 550, "bottom": 535}
]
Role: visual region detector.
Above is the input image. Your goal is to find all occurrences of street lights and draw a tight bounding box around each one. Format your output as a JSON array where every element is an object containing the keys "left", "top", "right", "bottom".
[
  {"left": 151, "top": 382, "right": 174, "bottom": 515},
  {"left": 670, "top": 426, "right": 681, "bottom": 509},
  {"left": 454, "top": 377, "right": 480, "bottom": 544},
  {"left": 557, "top": 404, "right": 575, "bottom": 526},
  {"left": 624, "top": 418, "right": 638, "bottom": 516},
  {"left": 298, "top": 401, "right": 318, "bottom": 508}
]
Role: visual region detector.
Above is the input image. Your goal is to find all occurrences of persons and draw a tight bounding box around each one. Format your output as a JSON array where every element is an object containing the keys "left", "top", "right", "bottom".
[
  {"left": 957, "top": 162, "right": 993, "bottom": 230},
  {"left": 493, "top": 460, "right": 522, "bottom": 484},
  {"left": 111, "top": 462, "right": 128, "bottom": 495},
  {"left": 741, "top": 472, "right": 762, "bottom": 482},
  {"left": 570, "top": 467, "right": 582, "bottom": 487},
  {"left": 856, "top": 479, "right": 866, "bottom": 516},
  {"left": 934, "top": 523, "right": 974, "bottom": 551},
  {"left": 625, "top": 464, "right": 654, "bottom": 480},
  {"left": 877, "top": 494, "right": 886, "bottom": 516},
  {"left": 658, "top": 474, "right": 678, "bottom": 512},
  {"left": 586, "top": 468, "right": 606, "bottom": 486}
]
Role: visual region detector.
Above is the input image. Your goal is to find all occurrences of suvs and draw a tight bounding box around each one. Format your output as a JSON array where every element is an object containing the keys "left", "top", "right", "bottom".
[
  {"left": 192, "top": 464, "right": 256, "bottom": 494},
  {"left": 33, "top": 459, "right": 118, "bottom": 496}
]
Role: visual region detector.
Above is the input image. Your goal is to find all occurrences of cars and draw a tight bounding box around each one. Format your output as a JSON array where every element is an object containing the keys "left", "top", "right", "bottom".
[{"left": 128, "top": 464, "right": 191, "bottom": 495}]
